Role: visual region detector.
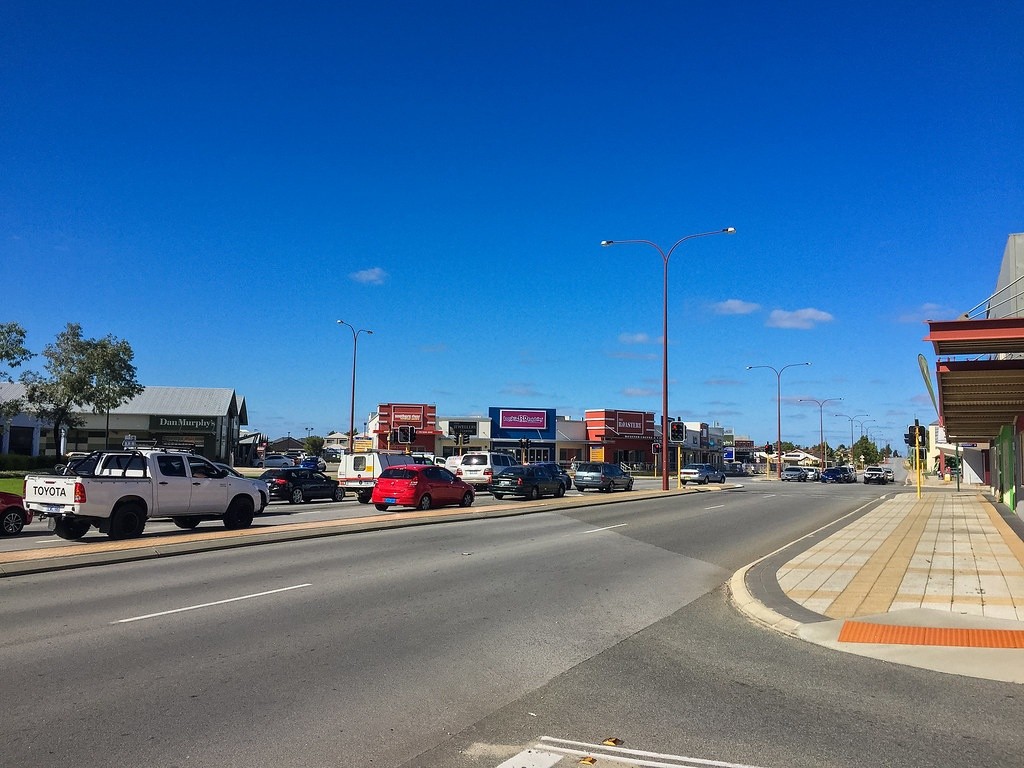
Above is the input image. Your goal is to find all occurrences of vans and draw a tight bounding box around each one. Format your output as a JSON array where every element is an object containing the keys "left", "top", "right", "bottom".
[{"left": 456, "top": 450, "right": 520, "bottom": 493}]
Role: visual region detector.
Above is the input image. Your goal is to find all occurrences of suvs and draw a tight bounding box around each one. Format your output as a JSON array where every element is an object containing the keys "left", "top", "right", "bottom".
[
  {"left": 573, "top": 462, "right": 634, "bottom": 493},
  {"left": 680, "top": 462, "right": 726, "bottom": 485}
]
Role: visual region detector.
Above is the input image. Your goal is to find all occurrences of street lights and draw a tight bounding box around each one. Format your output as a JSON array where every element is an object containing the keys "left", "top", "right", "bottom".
[
  {"left": 336, "top": 319, "right": 373, "bottom": 453},
  {"left": 746, "top": 362, "right": 812, "bottom": 480},
  {"left": 797, "top": 398, "right": 883, "bottom": 464},
  {"left": 600, "top": 227, "right": 737, "bottom": 493}
]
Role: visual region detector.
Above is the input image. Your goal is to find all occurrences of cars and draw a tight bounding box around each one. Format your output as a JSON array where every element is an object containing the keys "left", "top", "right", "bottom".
[
  {"left": 372, "top": 463, "right": 475, "bottom": 511},
  {"left": 0, "top": 490, "right": 33, "bottom": 537},
  {"left": 523, "top": 461, "right": 572, "bottom": 490},
  {"left": 47, "top": 451, "right": 252, "bottom": 528},
  {"left": 820, "top": 465, "right": 895, "bottom": 485},
  {"left": 253, "top": 455, "right": 295, "bottom": 468},
  {"left": 487, "top": 465, "right": 566, "bottom": 501},
  {"left": 258, "top": 467, "right": 346, "bottom": 505},
  {"left": 299, "top": 456, "right": 327, "bottom": 472},
  {"left": 802, "top": 467, "right": 819, "bottom": 482},
  {"left": 336, "top": 453, "right": 464, "bottom": 503},
  {"left": 780, "top": 466, "right": 807, "bottom": 482}
]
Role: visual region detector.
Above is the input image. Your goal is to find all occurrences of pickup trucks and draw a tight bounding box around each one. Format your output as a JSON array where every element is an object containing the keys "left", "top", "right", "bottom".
[{"left": 22, "top": 445, "right": 263, "bottom": 542}]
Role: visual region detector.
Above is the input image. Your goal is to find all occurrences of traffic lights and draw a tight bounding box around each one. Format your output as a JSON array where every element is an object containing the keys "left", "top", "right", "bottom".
[
  {"left": 518, "top": 438, "right": 532, "bottom": 449},
  {"left": 390, "top": 425, "right": 417, "bottom": 444},
  {"left": 453, "top": 432, "right": 470, "bottom": 446},
  {"left": 765, "top": 445, "right": 773, "bottom": 454},
  {"left": 652, "top": 443, "right": 660, "bottom": 454},
  {"left": 904, "top": 429, "right": 916, "bottom": 447},
  {"left": 670, "top": 421, "right": 685, "bottom": 443}
]
